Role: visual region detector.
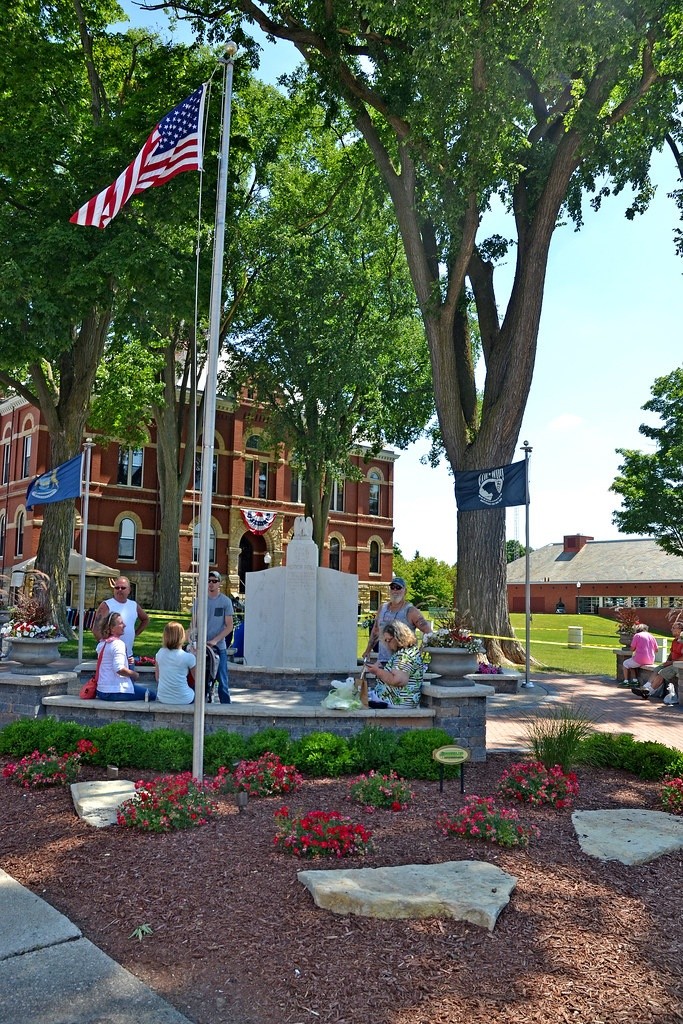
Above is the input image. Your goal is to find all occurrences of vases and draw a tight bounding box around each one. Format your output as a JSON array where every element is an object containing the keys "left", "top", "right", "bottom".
[
  {"left": 5, "top": 637, "right": 68, "bottom": 675},
  {"left": 424, "top": 646, "right": 486, "bottom": 686}
]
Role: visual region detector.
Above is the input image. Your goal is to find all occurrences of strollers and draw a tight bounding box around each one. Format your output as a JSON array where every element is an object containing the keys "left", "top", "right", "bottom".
[{"left": 182, "top": 641, "right": 221, "bottom": 703}]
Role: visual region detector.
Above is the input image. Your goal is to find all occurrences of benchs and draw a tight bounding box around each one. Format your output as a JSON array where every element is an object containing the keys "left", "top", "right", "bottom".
[{"left": 635, "top": 663, "right": 678, "bottom": 698}]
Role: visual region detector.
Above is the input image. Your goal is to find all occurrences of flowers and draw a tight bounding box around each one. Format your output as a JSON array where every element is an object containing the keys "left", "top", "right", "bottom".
[
  {"left": 1, "top": 619, "right": 62, "bottom": 639},
  {"left": 421, "top": 621, "right": 483, "bottom": 653}
]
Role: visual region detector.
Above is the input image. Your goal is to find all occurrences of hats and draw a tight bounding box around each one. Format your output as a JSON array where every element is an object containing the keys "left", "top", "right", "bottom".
[
  {"left": 389, "top": 577, "right": 405, "bottom": 588},
  {"left": 209, "top": 571, "right": 222, "bottom": 582}
]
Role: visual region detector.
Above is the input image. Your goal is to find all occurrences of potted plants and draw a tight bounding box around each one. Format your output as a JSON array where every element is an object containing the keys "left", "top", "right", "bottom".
[{"left": 617, "top": 607, "right": 639, "bottom": 652}]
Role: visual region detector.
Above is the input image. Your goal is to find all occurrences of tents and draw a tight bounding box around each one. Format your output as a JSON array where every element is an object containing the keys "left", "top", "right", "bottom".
[{"left": 7, "top": 547, "right": 120, "bottom": 609}]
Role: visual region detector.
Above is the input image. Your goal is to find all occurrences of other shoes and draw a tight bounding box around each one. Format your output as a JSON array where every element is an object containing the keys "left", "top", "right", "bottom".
[
  {"left": 632, "top": 688, "right": 651, "bottom": 696},
  {"left": 641, "top": 693, "right": 649, "bottom": 700},
  {"left": 618, "top": 682, "right": 629, "bottom": 687},
  {"left": 629, "top": 680, "right": 639, "bottom": 686}
]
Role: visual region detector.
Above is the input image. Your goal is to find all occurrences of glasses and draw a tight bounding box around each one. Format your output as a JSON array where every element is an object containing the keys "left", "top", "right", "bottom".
[
  {"left": 386, "top": 636, "right": 393, "bottom": 643},
  {"left": 209, "top": 579, "right": 219, "bottom": 583},
  {"left": 390, "top": 585, "right": 402, "bottom": 590},
  {"left": 115, "top": 587, "right": 126, "bottom": 590}
]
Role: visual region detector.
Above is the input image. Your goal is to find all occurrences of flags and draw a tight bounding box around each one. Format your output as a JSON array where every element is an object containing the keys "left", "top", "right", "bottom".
[
  {"left": 24, "top": 452, "right": 84, "bottom": 511},
  {"left": 453, "top": 457, "right": 530, "bottom": 512},
  {"left": 69, "top": 77, "right": 209, "bottom": 230}
]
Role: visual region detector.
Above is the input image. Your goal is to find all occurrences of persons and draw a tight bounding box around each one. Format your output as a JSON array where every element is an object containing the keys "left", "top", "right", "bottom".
[
  {"left": 94, "top": 612, "right": 157, "bottom": 700},
  {"left": 618, "top": 623, "right": 658, "bottom": 688},
  {"left": 631, "top": 622, "right": 683, "bottom": 699},
  {"left": 191, "top": 571, "right": 235, "bottom": 705},
  {"left": 361, "top": 577, "right": 432, "bottom": 663},
  {"left": 366, "top": 621, "right": 422, "bottom": 709},
  {"left": 91, "top": 575, "right": 150, "bottom": 684},
  {"left": 154, "top": 621, "right": 196, "bottom": 704}
]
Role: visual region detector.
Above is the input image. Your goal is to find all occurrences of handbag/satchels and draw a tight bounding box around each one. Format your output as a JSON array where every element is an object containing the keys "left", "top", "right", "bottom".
[
  {"left": 79, "top": 678, "right": 97, "bottom": 699},
  {"left": 355, "top": 657, "right": 368, "bottom": 709}
]
